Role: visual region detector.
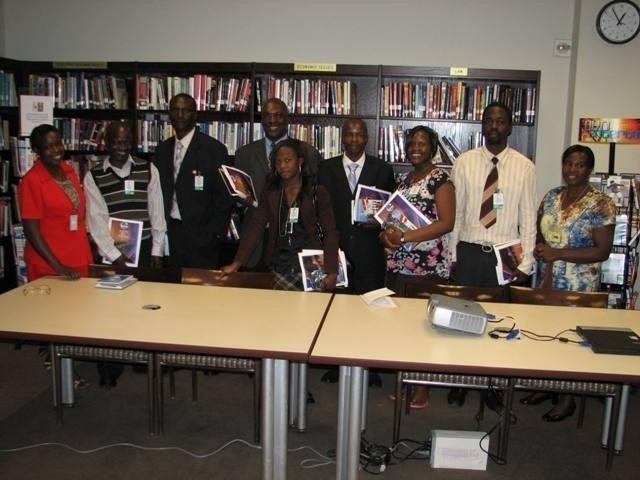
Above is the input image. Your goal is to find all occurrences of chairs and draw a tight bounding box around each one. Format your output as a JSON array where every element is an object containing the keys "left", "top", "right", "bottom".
[
  {"left": 154, "top": 267, "right": 275, "bottom": 445},
  {"left": 53, "top": 263, "right": 182, "bottom": 437},
  {"left": 393, "top": 277, "right": 509, "bottom": 465},
  {"left": 501, "top": 285, "right": 624, "bottom": 472}
]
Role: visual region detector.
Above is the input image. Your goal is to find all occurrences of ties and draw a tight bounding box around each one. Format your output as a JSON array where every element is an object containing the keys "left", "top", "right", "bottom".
[
  {"left": 347, "top": 165, "right": 359, "bottom": 194},
  {"left": 269, "top": 142, "right": 275, "bottom": 165},
  {"left": 478, "top": 155, "right": 497, "bottom": 229},
  {"left": 172, "top": 141, "right": 184, "bottom": 212}
]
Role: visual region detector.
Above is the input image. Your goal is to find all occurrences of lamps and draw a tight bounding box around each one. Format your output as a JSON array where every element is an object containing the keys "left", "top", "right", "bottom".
[{"left": 578, "top": 117, "right": 639, "bottom": 174}]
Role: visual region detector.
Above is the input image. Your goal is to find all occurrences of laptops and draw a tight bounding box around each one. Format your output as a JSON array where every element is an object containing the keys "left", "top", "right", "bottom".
[{"left": 576, "top": 325, "right": 640, "bottom": 355}]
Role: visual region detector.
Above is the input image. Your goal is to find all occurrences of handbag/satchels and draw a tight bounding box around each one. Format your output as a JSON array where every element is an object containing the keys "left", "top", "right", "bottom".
[{"left": 311, "top": 185, "right": 353, "bottom": 272}]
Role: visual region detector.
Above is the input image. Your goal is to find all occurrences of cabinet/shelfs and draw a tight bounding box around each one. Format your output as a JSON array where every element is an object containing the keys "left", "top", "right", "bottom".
[{"left": 587, "top": 176, "right": 639, "bottom": 310}]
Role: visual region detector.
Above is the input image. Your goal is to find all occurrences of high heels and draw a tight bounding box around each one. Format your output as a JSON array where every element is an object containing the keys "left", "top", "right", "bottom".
[
  {"left": 520, "top": 393, "right": 559, "bottom": 407},
  {"left": 39, "top": 346, "right": 90, "bottom": 390},
  {"left": 543, "top": 402, "right": 577, "bottom": 422}
]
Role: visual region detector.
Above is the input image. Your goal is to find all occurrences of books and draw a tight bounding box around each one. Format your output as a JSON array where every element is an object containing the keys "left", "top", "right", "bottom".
[
  {"left": 589, "top": 173, "right": 640, "bottom": 310},
  {"left": 0, "top": 68, "right": 536, "bottom": 278}
]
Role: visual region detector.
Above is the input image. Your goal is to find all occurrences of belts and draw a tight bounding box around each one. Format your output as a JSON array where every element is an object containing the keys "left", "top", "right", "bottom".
[{"left": 458, "top": 241, "right": 495, "bottom": 253}]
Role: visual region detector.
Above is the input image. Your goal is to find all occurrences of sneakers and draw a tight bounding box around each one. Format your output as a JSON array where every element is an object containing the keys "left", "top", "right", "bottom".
[
  {"left": 489, "top": 401, "right": 517, "bottom": 425},
  {"left": 322, "top": 370, "right": 430, "bottom": 409},
  {"left": 448, "top": 387, "right": 468, "bottom": 405}
]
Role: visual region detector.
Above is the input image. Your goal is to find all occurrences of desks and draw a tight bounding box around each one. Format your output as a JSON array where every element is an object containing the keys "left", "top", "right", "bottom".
[
  {"left": 309, "top": 293, "right": 640, "bottom": 479},
  {"left": 0, "top": 273, "right": 333, "bottom": 479}
]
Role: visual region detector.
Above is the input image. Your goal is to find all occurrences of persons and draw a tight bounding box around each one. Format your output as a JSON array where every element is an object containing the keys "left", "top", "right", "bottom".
[{"left": 18, "top": 92, "right": 617, "bottom": 422}]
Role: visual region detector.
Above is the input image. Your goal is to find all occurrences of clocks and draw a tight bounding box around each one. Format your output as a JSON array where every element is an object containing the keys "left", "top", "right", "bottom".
[{"left": 595, "top": 0, "right": 639, "bottom": 46}]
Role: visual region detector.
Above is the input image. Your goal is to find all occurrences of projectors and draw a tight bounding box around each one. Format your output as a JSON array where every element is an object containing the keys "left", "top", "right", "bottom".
[{"left": 426, "top": 293, "right": 488, "bottom": 336}]
